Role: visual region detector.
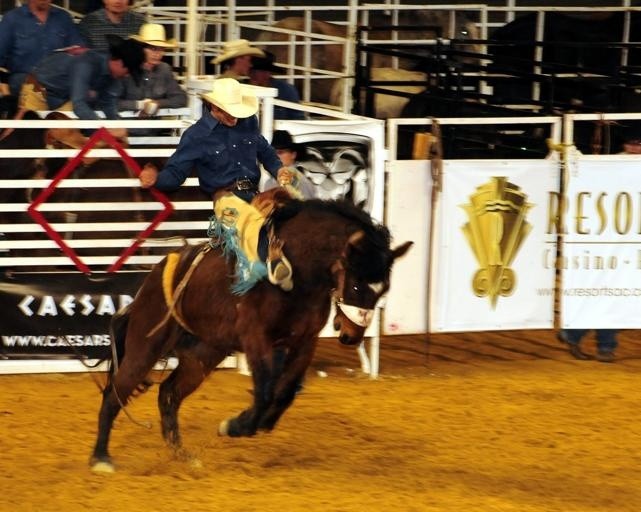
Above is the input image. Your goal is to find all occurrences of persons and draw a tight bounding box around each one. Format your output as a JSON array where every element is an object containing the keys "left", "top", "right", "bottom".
[
  {"left": 245, "top": 129, "right": 322, "bottom": 396},
  {"left": 138, "top": 76, "right": 299, "bottom": 292},
  {"left": 557, "top": 135, "right": 641, "bottom": 361}
]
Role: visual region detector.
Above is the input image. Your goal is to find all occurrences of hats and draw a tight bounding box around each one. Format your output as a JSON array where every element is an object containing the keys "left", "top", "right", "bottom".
[
  {"left": 209, "top": 38, "right": 285, "bottom": 76},
  {"left": 106, "top": 23, "right": 177, "bottom": 85},
  {"left": 198, "top": 77, "right": 259, "bottom": 119},
  {"left": 270, "top": 130, "right": 307, "bottom": 151}
]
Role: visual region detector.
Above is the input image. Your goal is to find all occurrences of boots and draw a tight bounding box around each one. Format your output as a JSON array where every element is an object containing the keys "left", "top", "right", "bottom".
[{"left": 265, "top": 251, "right": 294, "bottom": 292}]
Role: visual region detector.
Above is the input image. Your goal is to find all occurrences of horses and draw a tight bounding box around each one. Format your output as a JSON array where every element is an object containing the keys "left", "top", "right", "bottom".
[
  {"left": 253, "top": 11, "right": 482, "bottom": 121},
  {"left": 87, "top": 197, "right": 414, "bottom": 474}
]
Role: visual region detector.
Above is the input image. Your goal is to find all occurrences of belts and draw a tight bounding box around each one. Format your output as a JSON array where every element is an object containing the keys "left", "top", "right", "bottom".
[{"left": 225, "top": 178, "right": 258, "bottom": 191}]
